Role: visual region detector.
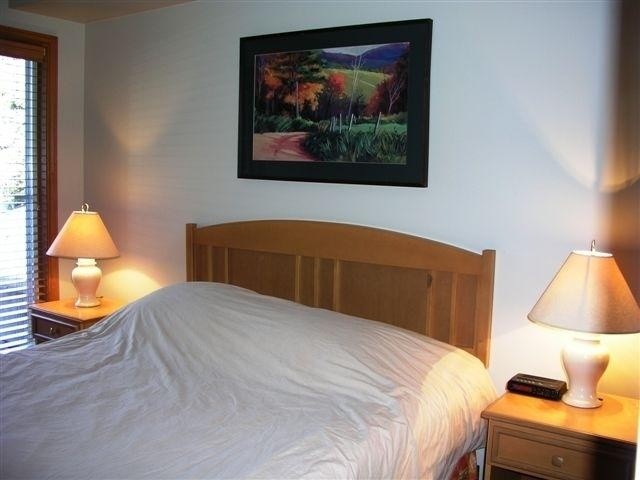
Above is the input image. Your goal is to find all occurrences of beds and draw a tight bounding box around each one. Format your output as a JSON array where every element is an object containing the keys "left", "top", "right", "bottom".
[{"left": 0, "top": 219, "right": 498, "bottom": 480}]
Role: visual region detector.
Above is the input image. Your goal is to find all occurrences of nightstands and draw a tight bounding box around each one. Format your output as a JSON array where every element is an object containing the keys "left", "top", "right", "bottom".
[
  {"left": 481, "top": 383, "right": 635, "bottom": 478},
  {"left": 25, "top": 298, "right": 120, "bottom": 345}
]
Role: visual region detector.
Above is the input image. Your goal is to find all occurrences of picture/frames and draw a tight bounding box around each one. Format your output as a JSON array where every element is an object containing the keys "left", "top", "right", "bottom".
[{"left": 235, "top": 17, "right": 435, "bottom": 190}]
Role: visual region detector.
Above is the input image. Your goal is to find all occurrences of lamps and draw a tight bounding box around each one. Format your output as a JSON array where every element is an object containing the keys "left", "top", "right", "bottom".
[
  {"left": 44, "top": 211, "right": 116, "bottom": 308},
  {"left": 529, "top": 249, "right": 637, "bottom": 408}
]
